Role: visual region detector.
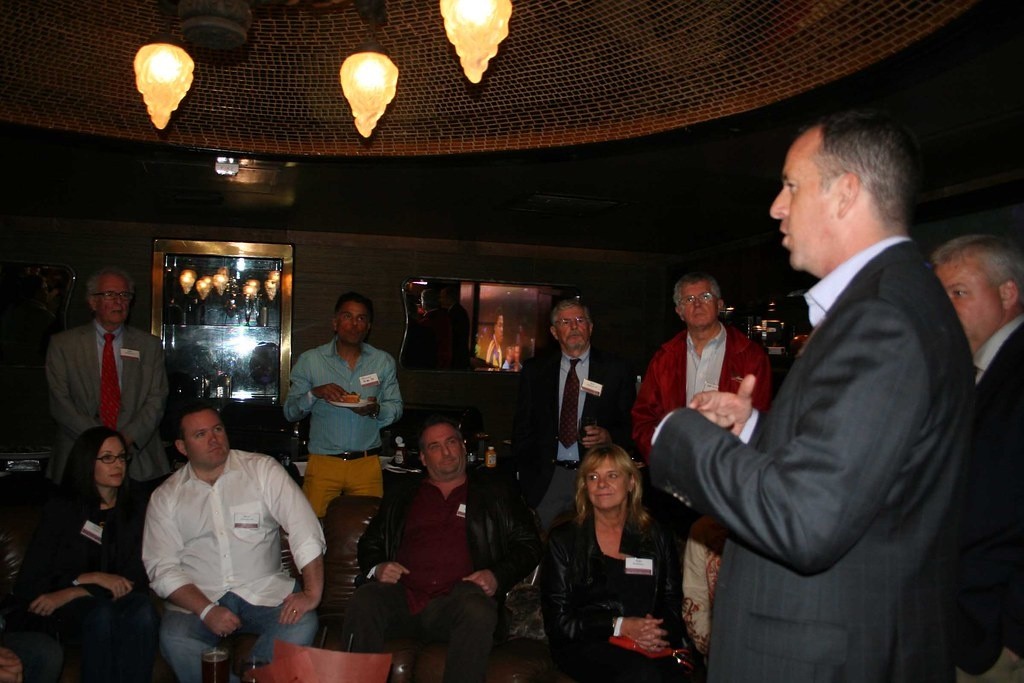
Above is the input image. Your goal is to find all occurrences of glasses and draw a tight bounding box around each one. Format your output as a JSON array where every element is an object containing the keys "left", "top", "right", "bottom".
[
  {"left": 96, "top": 453, "right": 133, "bottom": 464},
  {"left": 562, "top": 317, "right": 590, "bottom": 325},
  {"left": 93, "top": 291, "right": 134, "bottom": 298},
  {"left": 678, "top": 292, "right": 715, "bottom": 304}
]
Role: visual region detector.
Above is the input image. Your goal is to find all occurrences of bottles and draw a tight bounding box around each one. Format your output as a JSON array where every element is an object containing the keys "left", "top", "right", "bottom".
[
  {"left": 193, "top": 365, "right": 232, "bottom": 398},
  {"left": 395, "top": 436, "right": 407, "bottom": 465},
  {"left": 485, "top": 446, "right": 497, "bottom": 468}
]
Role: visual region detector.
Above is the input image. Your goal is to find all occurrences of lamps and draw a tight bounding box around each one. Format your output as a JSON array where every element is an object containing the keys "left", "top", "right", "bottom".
[
  {"left": 440, "top": 0, "right": 513, "bottom": 84},
  {"left": 134, "top": 0, "right": 194, "bottom": 129},
  {"left": 340, "top": 0, "right": 399, "bottom": 139},
  {"left": 179, "top": 257, "right": 281, "bottom": 301}
]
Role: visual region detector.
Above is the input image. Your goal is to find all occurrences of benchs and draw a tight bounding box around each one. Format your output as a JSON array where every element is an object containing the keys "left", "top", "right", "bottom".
[{"left": 1, "top": 468, "right": 573, "bottom": 683}]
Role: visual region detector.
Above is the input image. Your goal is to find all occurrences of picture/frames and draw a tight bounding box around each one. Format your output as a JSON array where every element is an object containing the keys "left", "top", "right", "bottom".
[{"left": 151, "top": 240, "right": 293, "bottom": 407}]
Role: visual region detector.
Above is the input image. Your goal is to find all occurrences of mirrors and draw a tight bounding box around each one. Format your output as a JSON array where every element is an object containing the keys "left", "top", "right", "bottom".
[{"left": 398, "top": 273, "right": 584, "bottom": 376}]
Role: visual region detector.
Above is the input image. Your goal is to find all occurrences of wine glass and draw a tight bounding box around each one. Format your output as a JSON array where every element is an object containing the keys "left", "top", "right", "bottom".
[{"left": 170, "top": 277, "right": 270, "bottom": 328}]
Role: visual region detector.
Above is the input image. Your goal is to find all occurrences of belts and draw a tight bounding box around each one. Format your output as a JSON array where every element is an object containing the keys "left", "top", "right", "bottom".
[
  {"left": 328, "top": 446, "right": 384, "bottom": 460},
  {"left": 557, "top": 460, "right": 579, "bottom": 469}
]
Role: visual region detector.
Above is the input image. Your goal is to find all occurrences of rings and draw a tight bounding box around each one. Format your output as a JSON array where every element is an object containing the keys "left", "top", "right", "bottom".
[{"left": 292, "top": 609, "right": 297, "bottom": 614}]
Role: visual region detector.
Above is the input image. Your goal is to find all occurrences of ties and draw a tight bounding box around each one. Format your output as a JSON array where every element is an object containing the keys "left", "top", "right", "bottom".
[
  {"left": 558, "top": 359, "right": 582, "bottom": 449},
  {"left": 101, "top": 333, "right": 121, "bottom": 431}
]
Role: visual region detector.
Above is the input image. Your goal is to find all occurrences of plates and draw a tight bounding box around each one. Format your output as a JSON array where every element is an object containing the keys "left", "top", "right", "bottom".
[{"left": 328, "top": 397, "right": 375, "bottom": 407}]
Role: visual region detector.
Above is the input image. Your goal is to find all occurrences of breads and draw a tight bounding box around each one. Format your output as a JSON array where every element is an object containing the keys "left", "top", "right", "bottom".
[{"left": 344, "top": 391, "right": 360, "bottom": 403}]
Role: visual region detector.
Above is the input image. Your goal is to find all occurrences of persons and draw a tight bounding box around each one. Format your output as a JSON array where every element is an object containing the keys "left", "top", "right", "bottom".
[
  {"left": 0, "top": 428, "right": 157, "bottom": 683},
  {"left": 43, "top": 266, "right": 173, "bottom": 512},
  {"left": 288, "top": 293, "right": 406, "bottom": 523},
  {"left": 633, "top": 274, "right": 769, "bottom": 468},
  {"left": 142, "top": 403, "right": 327, "bottom": 683},
  {"left": 516, "top": 297, "right": 637, "bottom": 510},
  {"left": 479, "top": 312, "right": 530, "bottom": 371},
  {"left": 647, "top": 108, "right": 1024, "bottom": 683},
  {"left": 539, "top": 444, "right": 698, "bottom": 683},
  {"left": 343, "top": 417, "right": 535, "bottom": 683}
]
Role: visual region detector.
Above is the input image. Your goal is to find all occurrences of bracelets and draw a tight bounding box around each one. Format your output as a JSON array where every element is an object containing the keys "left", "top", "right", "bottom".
[
  {"left": 199, "top": 601, "right": 219, "bottom": 622},
  {"left": 73, "top": 579, "right": 78, "bottom": 587},
  {"left": 613, "top": 617, "right": 623, "bottom": 639}
]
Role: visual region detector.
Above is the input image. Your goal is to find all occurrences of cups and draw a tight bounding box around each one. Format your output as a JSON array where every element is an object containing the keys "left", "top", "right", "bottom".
[
  {"left": 468, "top": 453, "right": 478, "bottom": 462},
  {"left": 201, "top": 646, "right": 230, "bottom": 683}
]
